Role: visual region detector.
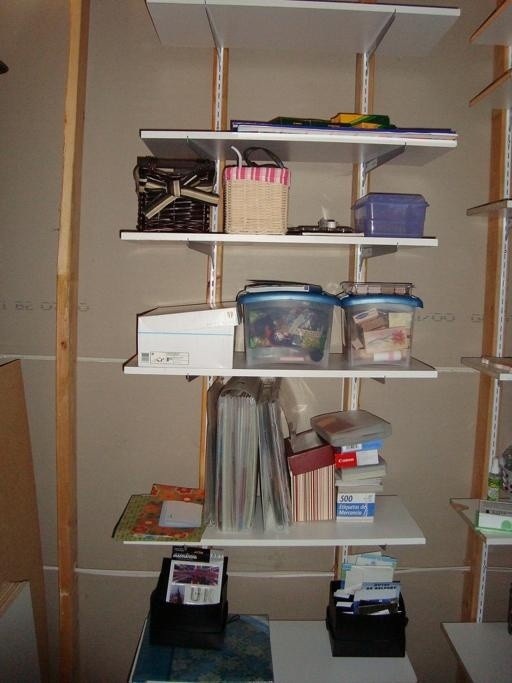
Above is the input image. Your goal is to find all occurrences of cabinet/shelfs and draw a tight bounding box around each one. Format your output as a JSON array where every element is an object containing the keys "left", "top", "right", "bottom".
[
  {"left": 441, "top": 0, "right": 512, "bottom": 682},
  {"left": 113, "top": 0, "right": 459, "bottom": 683}
]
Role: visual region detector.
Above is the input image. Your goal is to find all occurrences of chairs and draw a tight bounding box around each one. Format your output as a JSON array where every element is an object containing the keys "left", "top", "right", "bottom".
[{"left": 0, "top": 358, "right": 52, "bottom": 682}]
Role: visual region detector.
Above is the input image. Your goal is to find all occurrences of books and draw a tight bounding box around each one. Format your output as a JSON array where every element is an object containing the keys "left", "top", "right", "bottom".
[
  {"left": 311, "top": 410, "right": 392, "bottom": 493},
  {"left": 337, "top": 550, "right": 401, "bottom": 615},
  {"left": 244, "top": 278, "right": 322, "bottom": 293}
]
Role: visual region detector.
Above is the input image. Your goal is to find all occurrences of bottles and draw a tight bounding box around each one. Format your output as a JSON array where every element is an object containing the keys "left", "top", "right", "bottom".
[{"left": 487, "top": 457, "right": 501, "bottom": 501}]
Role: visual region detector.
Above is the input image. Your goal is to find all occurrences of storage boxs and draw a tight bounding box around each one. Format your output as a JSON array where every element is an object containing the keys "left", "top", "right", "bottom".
[
  {"left": 338, "top": 289, "right": 422, "bottom": 370},
  {"left": 236, "top": 287, "right": 343, "bottom": 369},
  {"left": 135, "top": 300, "right": 239, "bottom": 369},
  {"left": 326, "top": 582, "right": 406, "bottom": 656},
  {"left": 351, "top": 192, "right": 428, "bottom": 235},
  {"left": 149, "top": 556, "right": 229, "bottom": 650}
]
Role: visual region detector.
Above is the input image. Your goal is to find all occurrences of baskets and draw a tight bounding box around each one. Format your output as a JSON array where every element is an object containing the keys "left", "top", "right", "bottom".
[
  {"left": 224, "top": 145, "right": 289, "bottom": 235},
  {"left": 135, "top": 155, "right": 216, "bottom": 231}
]
